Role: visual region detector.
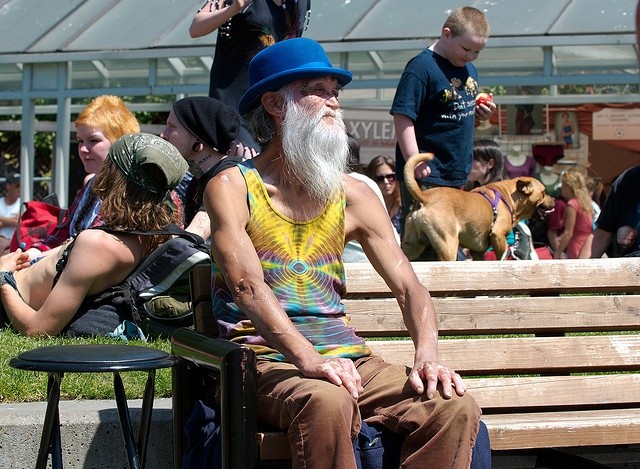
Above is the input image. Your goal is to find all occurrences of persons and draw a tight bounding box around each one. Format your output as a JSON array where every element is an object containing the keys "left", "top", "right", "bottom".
[
  {"left": 366, "top": 158, "right": 404, "bottom": 245},
  {"left": 343, "top": 133, "right": 361, "bottom": 172},
  {"left": 204, "top": 36, "right": 482, "bottom": 468},
  {"left": 160, "top": 96, "right": 240, "bottom": 242},
  {"left": 390, "top": 6, "right": 487, "bottom": 260},
  {"left": 559, "top": 110, "right": 575, "bottom": 146},
  {"left": 554, "top": 167, "right": 593, "bottom": 259},
  {"left": 505, "top": 146, "right": 536, "bottom": 179},
  {"left": 466, "top": 141, "right": 505, "bottom": 188},
  {"left": 190, "top": 0, "right": 311, "bottom": 107},
  {"left": 581, "top": 169, "right": 603, "bottom": 205},
  {"left": 548, "top": 199, "right": 566, "bottom": 252},
  {"left": 532, "top": 164, "right": 565, "bottom": 202},
  {"left": 0, "top": 173, "right": 22, "bottom": 253},
  {"left": 579, "top": 162, "right": 639, "bottom": 259},
  {"left": 21, "top": 93, "right": 182, "bottom": 263},
  {"left": 1, "top": 132, "right": 188, "bottom": 342}
]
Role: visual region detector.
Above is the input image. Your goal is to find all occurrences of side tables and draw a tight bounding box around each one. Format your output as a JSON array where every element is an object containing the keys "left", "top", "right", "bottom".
[{"left": 7, "top": 345, "right": 179, "bottom": 468}]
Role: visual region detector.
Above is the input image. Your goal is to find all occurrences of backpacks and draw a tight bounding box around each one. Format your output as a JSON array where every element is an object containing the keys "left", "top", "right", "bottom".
[
  {"left": 9, "top": 201, "right": 70, "bottom": 253},
  {"left": 51, "top": 226, "right": 213, "bottom": 337}
]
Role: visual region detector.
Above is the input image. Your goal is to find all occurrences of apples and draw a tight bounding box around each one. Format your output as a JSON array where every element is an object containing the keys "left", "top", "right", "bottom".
[{"left": 474, "top": 92, "right": 491, "bottom": 111}]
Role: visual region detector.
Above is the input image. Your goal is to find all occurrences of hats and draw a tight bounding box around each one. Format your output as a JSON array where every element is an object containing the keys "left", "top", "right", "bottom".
[
  {"left": 6, "top": 171, "right": 20, "bottom": 183},
  {"left": 172, "top": 96, "right": 240, "bottom": 152},
  {"left": 239, "top": 38, "right": 353, "bottom": 122}
]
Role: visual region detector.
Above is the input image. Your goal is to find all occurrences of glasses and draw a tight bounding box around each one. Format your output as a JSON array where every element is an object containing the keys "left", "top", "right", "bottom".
[{"left": 373, "top": 172, "right": 396, "bottom": 183}]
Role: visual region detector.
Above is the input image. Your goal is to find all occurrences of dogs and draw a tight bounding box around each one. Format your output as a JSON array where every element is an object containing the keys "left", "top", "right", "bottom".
[{"left": 401, "top": 152, "right": 556, "bottom": 263}]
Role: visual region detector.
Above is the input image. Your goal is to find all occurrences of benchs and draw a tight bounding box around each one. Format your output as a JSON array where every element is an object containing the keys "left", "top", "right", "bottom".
[{"left": 169, "top": 258, "right": 640, "bottom": 468}]
